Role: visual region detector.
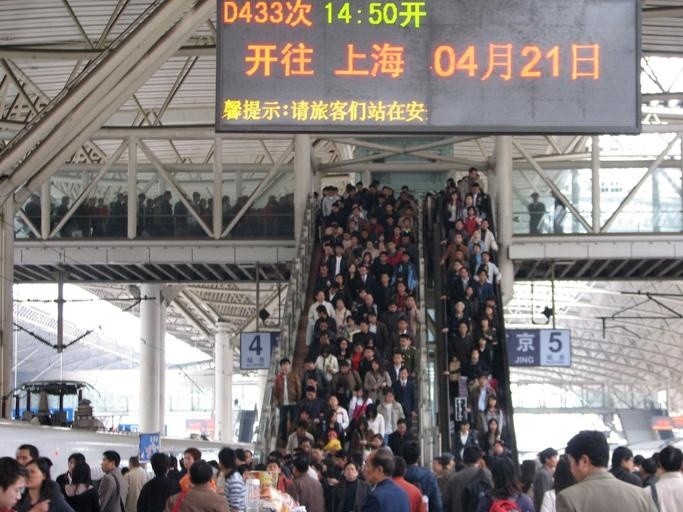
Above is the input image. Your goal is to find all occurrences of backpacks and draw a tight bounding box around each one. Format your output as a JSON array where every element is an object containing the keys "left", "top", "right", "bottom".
[{"left": 487, "top": 490, "right": 522, "bottom": 512}]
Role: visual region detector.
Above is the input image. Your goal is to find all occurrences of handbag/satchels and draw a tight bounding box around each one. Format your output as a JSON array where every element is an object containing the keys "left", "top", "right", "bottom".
[{"left": 463, "top": 473, "right": 492, "bottom": 512}]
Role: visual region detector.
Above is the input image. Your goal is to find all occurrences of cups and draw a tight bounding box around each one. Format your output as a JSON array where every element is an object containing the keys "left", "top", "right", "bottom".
[{"left": 244, "top": 477, "right": 260, "bottom": 511}]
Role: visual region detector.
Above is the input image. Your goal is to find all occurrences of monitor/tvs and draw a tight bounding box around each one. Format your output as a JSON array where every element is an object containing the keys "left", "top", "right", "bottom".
[{"left": 139, "top": 434, "right": 160, "bottom": 464}]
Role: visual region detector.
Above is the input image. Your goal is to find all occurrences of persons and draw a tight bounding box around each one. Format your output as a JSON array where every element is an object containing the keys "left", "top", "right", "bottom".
[
  {"left": 25, "top": 193, "right": 280, "bottom": 239},
  {"left": 1, "top": 430, "right": 683, "bottom": 512},
  {"left": 273, "top": 168, "right": 514, "bottom": 462},
  {"left": 528, "top": 189, "right": 564, "bottom": 234}
]
